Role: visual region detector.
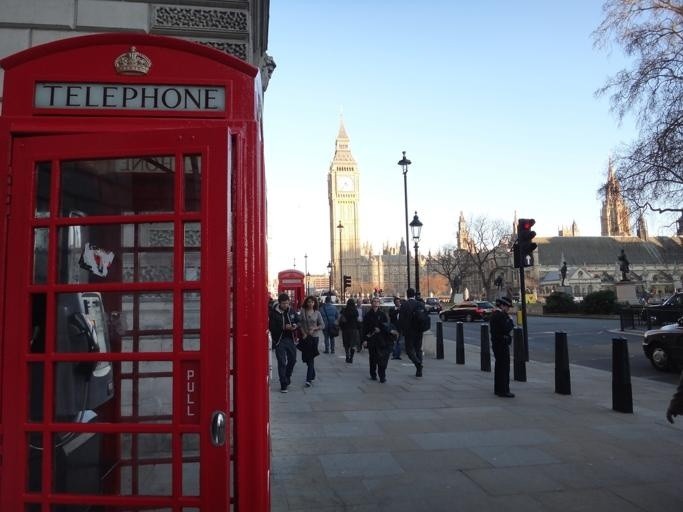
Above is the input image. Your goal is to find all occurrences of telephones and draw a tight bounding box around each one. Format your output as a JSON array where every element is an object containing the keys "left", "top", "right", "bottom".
[{"left": 54, "top": 292, "right": 115, "bottom": 417}]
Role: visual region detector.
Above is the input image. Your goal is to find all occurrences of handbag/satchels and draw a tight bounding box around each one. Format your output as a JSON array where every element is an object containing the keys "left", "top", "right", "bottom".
[
  {"left": 327, "top": 323, "right": 339, "bottom": 337},
  {"left": 492, "top": 329, "right": 511, "bottom": 347},
  {"left": 412, "top": 310, "right": 431, "bottom": 334}
]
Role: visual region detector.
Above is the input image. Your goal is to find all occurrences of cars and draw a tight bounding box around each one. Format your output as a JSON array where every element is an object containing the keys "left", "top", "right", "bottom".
[{"left": 378, "top": 297, "right": 394, "bottom": 306}]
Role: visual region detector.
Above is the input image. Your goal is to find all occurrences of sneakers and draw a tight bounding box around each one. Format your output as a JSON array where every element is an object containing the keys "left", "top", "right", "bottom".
[
  {"left": 286, "top": 376, "right": 290, "bottom": 385},
  {"left": 370, "top": 375, "right": 386, "bottom": 384},
  {"left": 305, "top": 381, "right": 311, "bottom": 386},
  {"left": 416, "top": 365, "right": 422, "bottom": 377},
  {"left": 281, "top": 383, "right": 287, "bottom": 393}
]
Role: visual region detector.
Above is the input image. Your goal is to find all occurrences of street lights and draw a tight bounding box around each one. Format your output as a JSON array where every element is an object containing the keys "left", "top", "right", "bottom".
[
  {"left": 397, "top": 151, "right": 413, "bottom": 300},
  {"left": 304, "top": 253, "right": 310, "bottom": 296},
  {"left": 326, "top": 261, "right": 332, "bottom": 290},
  {"left": 409, "top": 210, "right": 422, "bottom": 299},
  {"left": 337, "top": 220, "right": 343, "bottom": 301}
]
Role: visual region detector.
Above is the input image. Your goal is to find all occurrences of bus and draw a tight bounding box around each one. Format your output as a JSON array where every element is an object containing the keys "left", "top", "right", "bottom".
[{"left": 318, "top": 289, "right": 335, "bottom": 303}]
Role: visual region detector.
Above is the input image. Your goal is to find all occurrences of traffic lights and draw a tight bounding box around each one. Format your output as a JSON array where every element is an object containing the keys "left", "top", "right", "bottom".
[{"left": 519, "top": 219, "right": 537, "bottom": 266}]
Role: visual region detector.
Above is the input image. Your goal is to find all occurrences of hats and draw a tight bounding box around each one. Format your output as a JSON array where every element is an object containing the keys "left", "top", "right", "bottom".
[{"left": 496, "top": 297, "right": 514, "bottom": 307}]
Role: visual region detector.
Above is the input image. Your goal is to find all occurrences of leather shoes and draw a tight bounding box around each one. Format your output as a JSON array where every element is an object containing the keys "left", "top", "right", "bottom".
[{"left": 504, "top": 392, "right": 514, "bottom": 397}]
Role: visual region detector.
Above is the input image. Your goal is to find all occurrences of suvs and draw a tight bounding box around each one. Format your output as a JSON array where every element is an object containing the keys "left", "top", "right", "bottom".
[
  {"left": 345, "top": 276, "right": 350, "bottom": 287},
  {"left": 423, "top": 297, "right": 441, "bottom": 314},
  {"left": 643, "top": 317, "right": 683, "bottom": 370},
  {"left": 438, "top": 301, "right": 496, "bottom": 322},
  {"left": 639, "top": 292, "right": 683, "bottom": 323}
]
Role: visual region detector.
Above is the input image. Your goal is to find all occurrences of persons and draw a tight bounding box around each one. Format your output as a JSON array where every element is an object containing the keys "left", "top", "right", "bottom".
[
  {"left": 617, "top": 249, "right": 629, "bottom": 281},
  {"left": 489, "top": 296, "right": 516, "bottom": 397},
  {"left": 266, "top": 286, "right": 428, "bottom": 395},
  {"left": 495, "top": 271, "right": 504, "bottom": 292},
  {"left": 644, "top": 287, "right": 656, "bottom": 305},
  {"left": 666, "top": 373, "right": 683, "bottom": 425},
  {"left": 560, "top": 260, "right": 568, "bottom": 286}
]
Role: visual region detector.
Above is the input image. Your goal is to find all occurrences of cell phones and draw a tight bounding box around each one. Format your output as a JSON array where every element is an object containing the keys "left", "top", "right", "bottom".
[{"left": 292, "top": 324, "right": 296, "bottom": 328}]
